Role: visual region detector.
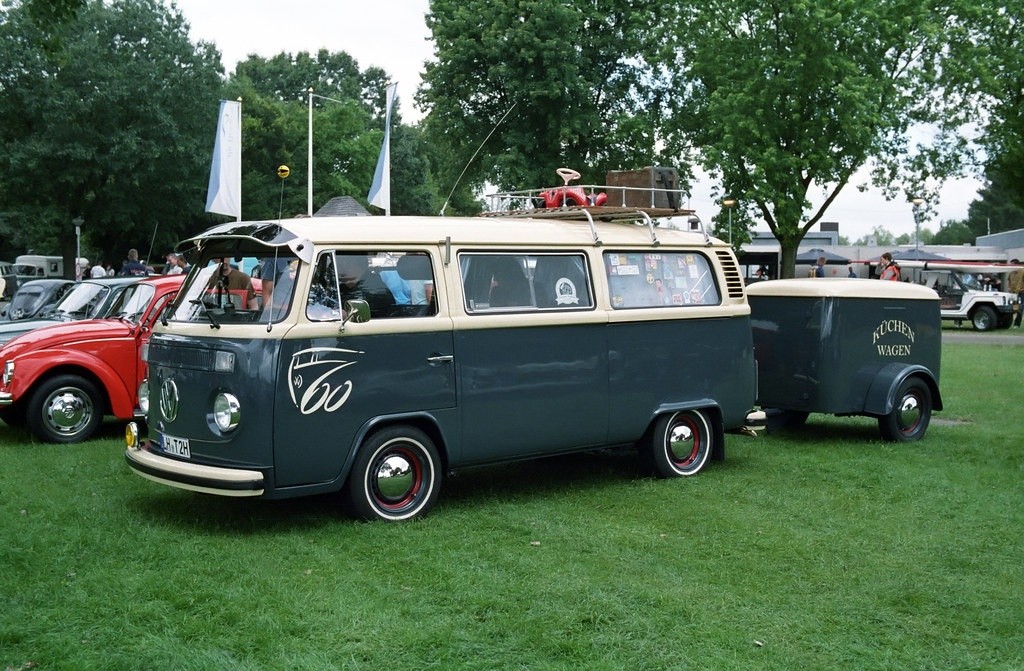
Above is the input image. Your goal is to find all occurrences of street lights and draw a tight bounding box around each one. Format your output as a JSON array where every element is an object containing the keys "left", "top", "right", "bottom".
[
  {"left": 724, "top": 201, "right": 734, "bottom": 244},
  {"left": 912, "top": 199, "right": 924, "bottom": 261},
  {"left": 72, "top": 218, "right": 85, "bottom": 280}
]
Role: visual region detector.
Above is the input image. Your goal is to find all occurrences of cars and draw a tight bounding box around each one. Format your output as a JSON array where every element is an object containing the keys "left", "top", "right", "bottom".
[{"left": 0, "top": 254, "right": 262, "bottom": 443}]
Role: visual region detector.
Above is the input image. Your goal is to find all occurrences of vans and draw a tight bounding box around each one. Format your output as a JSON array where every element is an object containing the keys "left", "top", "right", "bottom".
[{"left": 124, "top": 167, "right": 757, "bottom": 525}]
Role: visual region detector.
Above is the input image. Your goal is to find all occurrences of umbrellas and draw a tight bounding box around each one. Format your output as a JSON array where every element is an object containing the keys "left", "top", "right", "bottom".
[
  {"left": 863, "top": 249, "right": 952, "bottom": 265},
  {"left": 779, "top": 248, "right": 851, "bottom": 266}
]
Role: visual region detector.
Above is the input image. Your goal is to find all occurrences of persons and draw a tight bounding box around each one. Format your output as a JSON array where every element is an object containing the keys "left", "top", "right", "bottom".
[
  {"left": 91, "top": 249, "right": 149, "bottom": 279},
  {"left": 848, "top": 267, "right": 857, "bottom": 278},
  {"left": 166, "top": 252, "right": 190, "bottom": 275},
  {"left": 875, "top": 252, "right": 901, "bottom": 281},
  {"left": 756, "top": 265, "right": 768, "bottom": 279},
  {"left": 82, "top": 263, "right": 91, "bottom": 280},
  {"left": 335, "top": 250, "right": 396, "bottom": 308},
  {"left": 260, "top": 258, "right": 290, "bottom": 319},
  {"left": 808, "top": 256, "right": 826, "bottom": 277},
  {"left": 202, "top": 257, "right": 255, "bottom": 307},
  {"left": 379, "top": 251, "right": 433, "bottom": 305},
  {"left": 958, "top": 271, "right": 977, "bottom": 289},
  {"left": 1007, "top": 258, "right": 1024, "bottom": 330}
]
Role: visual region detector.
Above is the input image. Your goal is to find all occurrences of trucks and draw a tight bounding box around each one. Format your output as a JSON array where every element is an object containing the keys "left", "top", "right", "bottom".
[{"left": 869, "top": 257, "right": 1022, "bottom": 331}]
